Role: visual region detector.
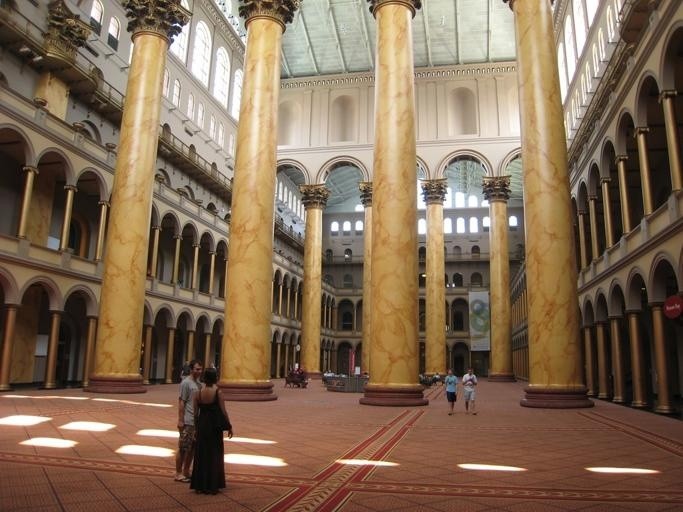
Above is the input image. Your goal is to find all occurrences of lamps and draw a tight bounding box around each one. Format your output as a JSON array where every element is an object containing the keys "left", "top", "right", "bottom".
[{"left": 218, "top": 0, "right": 246, "bottom": 37}]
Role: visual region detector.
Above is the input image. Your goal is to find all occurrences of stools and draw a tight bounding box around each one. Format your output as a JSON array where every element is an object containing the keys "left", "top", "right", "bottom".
[{"left": 420, "top": 375, "right": 444, "bottom": 386}]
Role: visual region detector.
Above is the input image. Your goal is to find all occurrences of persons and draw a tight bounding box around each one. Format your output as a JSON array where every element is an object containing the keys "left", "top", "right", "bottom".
[
  {"left": 190, "top": 366, "right": 233, "bottom": 495},
  {"left": 174, "top": 359, "right": 203, "bottom": 484},
  {"left": 361, "top": 372, "right": 369, "bottom": 379},
  {"left": 285, "top": 368, "right": 307, "bottom": 388},
  {"left": 418, "top": 372, "right": 440, "bottom": 386},
  {"left": 444, "top": 367, "right": 459, "bottom": 416},
  {"left": 323, "top": 370, "right": 346, "bottom": 383},
  {"left": 461, "top": 367, "right": 477, "bottom": 415},
  {"left": 180, "top": 360, "right": 190, "bottom": 380}
]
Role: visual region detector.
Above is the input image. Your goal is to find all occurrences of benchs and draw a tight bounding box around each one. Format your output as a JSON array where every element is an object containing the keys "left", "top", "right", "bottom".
[{"left": 285, "top": 375, "right": 302, "bottom": 387}]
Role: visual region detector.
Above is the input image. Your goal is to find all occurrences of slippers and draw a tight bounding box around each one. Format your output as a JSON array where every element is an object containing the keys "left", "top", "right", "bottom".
[{"left": 175, "top": 476, "right": 191, "bottom": 483}]
